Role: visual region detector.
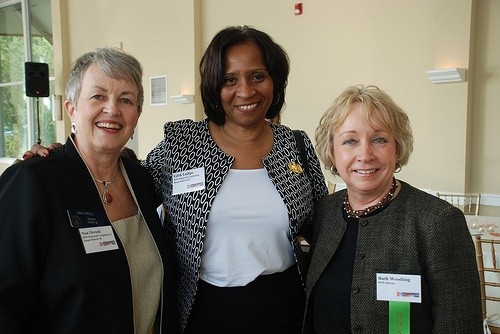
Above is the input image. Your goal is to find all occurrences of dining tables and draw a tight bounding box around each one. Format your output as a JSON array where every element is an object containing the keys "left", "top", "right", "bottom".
[{"left": 464, "top": 215, "right": 500, "bottom": 319}]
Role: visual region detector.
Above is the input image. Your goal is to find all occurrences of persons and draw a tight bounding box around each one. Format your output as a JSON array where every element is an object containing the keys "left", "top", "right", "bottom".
[
  {"left": 300, "top": 84, "right": 484, "bottom": 334},
  {"left": 0, "top": 25, "right": 330, "bottom": 334}
]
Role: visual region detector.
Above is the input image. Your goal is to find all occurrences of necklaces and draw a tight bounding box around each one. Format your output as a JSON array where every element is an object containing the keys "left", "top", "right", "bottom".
[
  {"left": 343, "top": 176, "right": 399, "bottom": 218},
  {"left": 93, "top": 167, "right": 119, "bottom": 206}
]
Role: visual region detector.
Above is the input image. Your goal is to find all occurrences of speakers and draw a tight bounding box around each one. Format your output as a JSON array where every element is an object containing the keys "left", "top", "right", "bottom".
[{"left": 24, "top": 62, "right": 50, "bottom": 98}]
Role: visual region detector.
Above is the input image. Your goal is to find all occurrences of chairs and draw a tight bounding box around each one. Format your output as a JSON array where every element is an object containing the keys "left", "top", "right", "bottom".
[
  {"left": 475, "top": 236, "right": 500, "bottom": 334},
  {"left": 437, "top": 193, "right": 481, "bottom": 217}
]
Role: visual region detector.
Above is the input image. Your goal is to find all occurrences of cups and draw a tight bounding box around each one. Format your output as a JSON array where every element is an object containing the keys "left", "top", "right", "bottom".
[{"left": 472, "top": 223, "right": 500, "bottom": 234}]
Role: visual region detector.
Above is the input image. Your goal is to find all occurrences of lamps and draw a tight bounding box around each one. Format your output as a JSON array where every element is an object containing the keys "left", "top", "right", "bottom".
[
  {"left": 170, "top": 94, "right": 195, "bottom": 103},
  {"left": 425, "top": 67, "right": 467, "bottom": 86}
]
